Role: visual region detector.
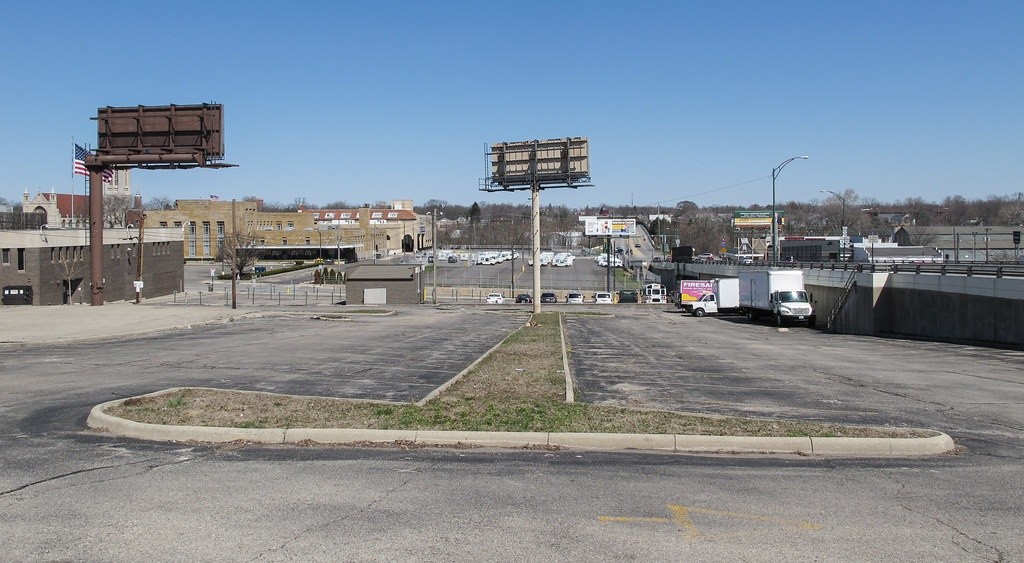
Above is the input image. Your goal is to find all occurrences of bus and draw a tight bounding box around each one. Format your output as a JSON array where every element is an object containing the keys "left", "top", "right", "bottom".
[{"left": 640, "top": 283, "right": 667, "bottom": 304}]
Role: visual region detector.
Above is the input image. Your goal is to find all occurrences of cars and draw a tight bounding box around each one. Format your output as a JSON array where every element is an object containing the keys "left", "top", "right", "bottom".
[
  {"left": 184, "top": 258, "right": 186, "bottom": 264},
  {"left": 616, "top": 246, "right": 621, "bottom": 249},
  {"left": 415, "top": 255, "right": 423, "bottom": 261},
  {"left": 591, "top": 291, "right": 614, "bottom": 304},
  {"left": 486, "top": 293, "right": 505, "bottom": 304},
  {"left": 527, "top": 251, "right": 577, "bottom": 267},
  {"left": 594, "top": 253, "right": 623, "bottom": 267},
  {"left": 565, "top": 293, "right": 586, "bottom": 304},
  {"left": 438, "top": 250, "right": 519, "bottom": 265},
  {"left": 618, "top": 248, "right": 623, "bottom": 253},
  {"left": 616, "top": 290, "right": 639, "bottom": 303},
  {"left": 666, "top": 255, "right": 672, "bottom": 262},
  {"left": 635, "top": 244, "right": 641, "bottom": 247},
  {"left": 428, "top": 256, "right": 434, "bottom": 263},
  {"left": 422, "top": 252, "right": 429, "bottom": 259},
  {"left": 427, "top": 249, "right": 438, "bottom": 256},
  {"left": 615, "top": 248, "right": 618, "bottom": 253},
  {"left": 515, "top": 294, "right": 533, "bottom": 303},
  {"left": 654, "top": 257, "right": 661, "bottom": 262},
  {"left": 782, "top": 256, "right": 798, "bottom": 263},
  {"left": 541, "top": 293, "right": 558, "bottom": 303}
]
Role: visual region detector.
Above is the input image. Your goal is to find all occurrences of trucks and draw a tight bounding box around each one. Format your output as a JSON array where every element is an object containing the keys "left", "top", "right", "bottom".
[
  {"left": 685, "top": 278, "right": 740, "bottom": 317},
  {"left": 738, "top": 270, "right": 817, "bottom": 327},
  {"left": 671, "top": 245, "right": 693, "bottom": 264}
]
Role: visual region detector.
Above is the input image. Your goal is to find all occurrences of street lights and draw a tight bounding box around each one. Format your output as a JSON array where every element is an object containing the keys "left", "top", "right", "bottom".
[
  {"left": 182, "top": 221, "right": 191, "bottom": 231},
  {"left": 772, "top": 155, "right": 808, "bottom": 267},
  {"left": 426, "top": 209, "right": 443, "bottom": 303},
  {"left": 820, "top": 189, "right": 846, "bottom": 237},
  {"left": 314, "top": 229, "right": 321, "bottom": 263}
]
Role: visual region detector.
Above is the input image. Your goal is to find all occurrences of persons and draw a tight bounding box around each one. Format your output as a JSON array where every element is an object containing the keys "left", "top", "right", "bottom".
[{"left": 237, "top": 274, "right": 240, "bottom": 284}]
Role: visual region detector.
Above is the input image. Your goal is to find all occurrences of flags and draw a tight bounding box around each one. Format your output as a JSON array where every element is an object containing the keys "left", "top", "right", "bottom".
[{"left": 74, "top": 143, "right": 113, "bottom": 183}]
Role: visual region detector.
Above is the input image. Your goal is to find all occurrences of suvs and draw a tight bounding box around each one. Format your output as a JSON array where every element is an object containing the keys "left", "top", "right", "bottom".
[{"left": 698, "top": 253, "right": 713, "bottom": 259}]
